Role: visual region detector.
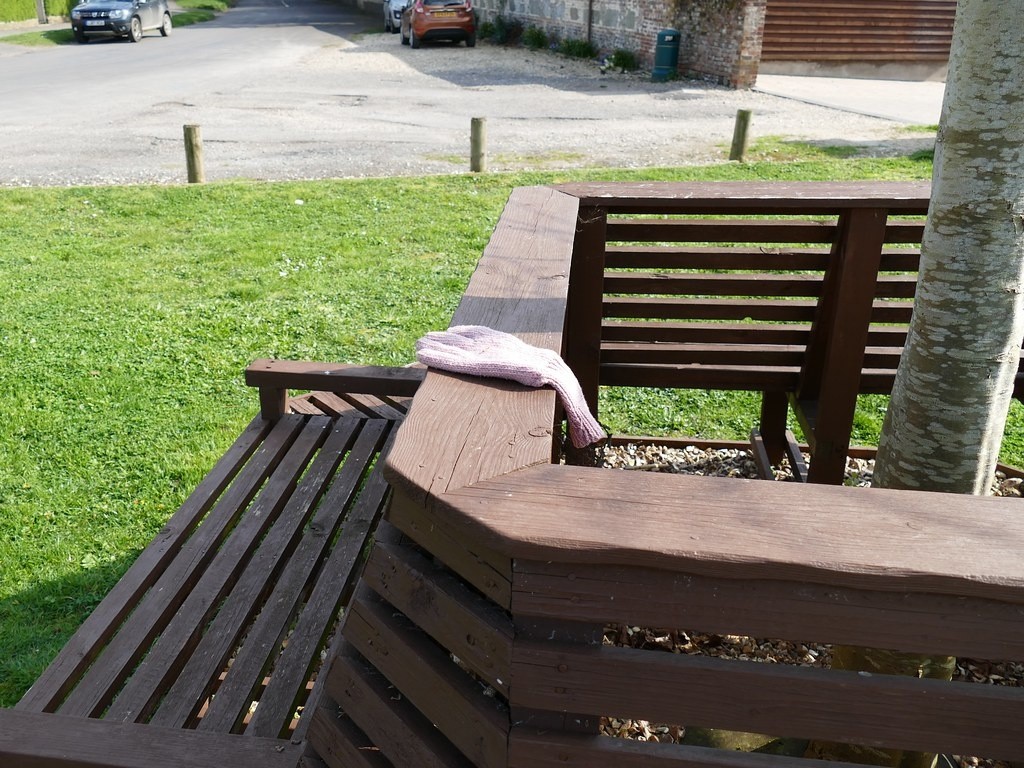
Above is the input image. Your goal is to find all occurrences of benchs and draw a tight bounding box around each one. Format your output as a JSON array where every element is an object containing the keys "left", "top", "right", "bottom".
[
  {"left": 555, "top": 180, "right": 932, "bottom": 484},
  {"left": 0, "top": 187, "right": 579, "bottom": 768}
]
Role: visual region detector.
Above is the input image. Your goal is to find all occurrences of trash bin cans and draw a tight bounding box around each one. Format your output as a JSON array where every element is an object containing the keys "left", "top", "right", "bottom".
[{"left": 651, "top": 28, "right": 680, "bottom": 82}]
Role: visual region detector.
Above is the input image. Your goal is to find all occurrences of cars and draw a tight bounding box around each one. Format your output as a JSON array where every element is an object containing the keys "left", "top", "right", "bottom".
[{"left": 382, "top": 0, "right": 416, "bottom": 34}]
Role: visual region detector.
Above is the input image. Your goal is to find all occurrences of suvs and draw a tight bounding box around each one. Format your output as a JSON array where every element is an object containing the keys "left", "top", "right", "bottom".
[
  {"left": 401, "top": 0, "right": 477, "bottom": 49},
  {"left": 69, "top": 0, "right": 172, "bottom": 44}
]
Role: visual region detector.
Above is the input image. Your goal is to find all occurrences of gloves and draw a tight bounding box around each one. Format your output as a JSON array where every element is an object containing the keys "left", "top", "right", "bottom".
[{"left": 414, "top": 324, "right": 608, "bottom": 450}]
